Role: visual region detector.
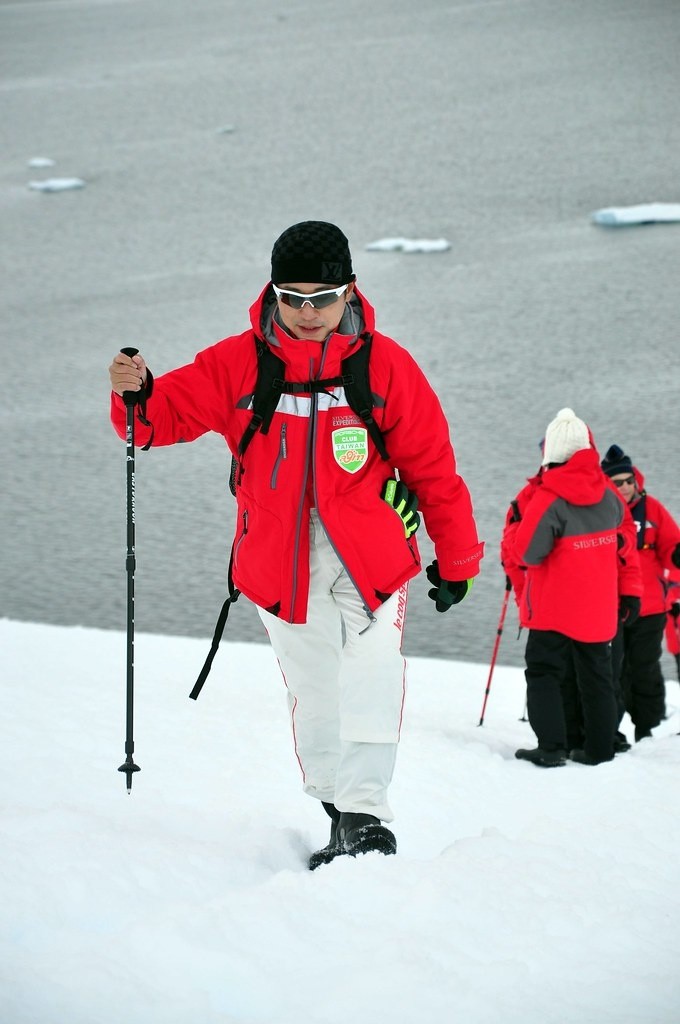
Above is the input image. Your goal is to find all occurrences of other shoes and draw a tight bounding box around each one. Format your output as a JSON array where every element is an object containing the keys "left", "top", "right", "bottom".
[
  {"left": 515, "top": 748, "right": 567, "bottom": 768},
  {"left": 309, "top": 843, "right": 346, "bottom": 870},
  {"left": 343, "top": 823, "right": 398, "bottom": 855},
  {"left": 569, "top": 749, "right": 593, "bottom": 764}
]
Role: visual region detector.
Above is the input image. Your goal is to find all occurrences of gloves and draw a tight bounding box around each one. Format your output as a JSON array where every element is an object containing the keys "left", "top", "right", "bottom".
[{"left": 426, "top": 559, "right": 468, "bottom": 613}]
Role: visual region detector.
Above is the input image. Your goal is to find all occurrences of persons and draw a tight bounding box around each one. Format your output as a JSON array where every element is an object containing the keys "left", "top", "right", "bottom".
[
  {"left": 110, "top": 221, "right": 485, "bottom": 870},
  {"left": 500, "top": 407, "right": 680, "bottom": 768}
]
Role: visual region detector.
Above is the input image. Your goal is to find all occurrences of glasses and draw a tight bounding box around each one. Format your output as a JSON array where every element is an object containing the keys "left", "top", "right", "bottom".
[
  {"left": 272, "top": 284, "right": 349, "bottom": 310},
  {"left": 613, "top": 475, "right": 635, "bottom": 486}
]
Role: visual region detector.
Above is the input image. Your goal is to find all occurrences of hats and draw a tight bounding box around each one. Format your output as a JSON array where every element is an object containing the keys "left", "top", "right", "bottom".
[
  {"left": 541, "top": 407, "right": 590, "bottom": 466},
  {"left": 601, "top": 444, "right": 634, "bottom": 477},
  {"left": 271, "top": 220, "right": 356, "bottom": 284}
]
